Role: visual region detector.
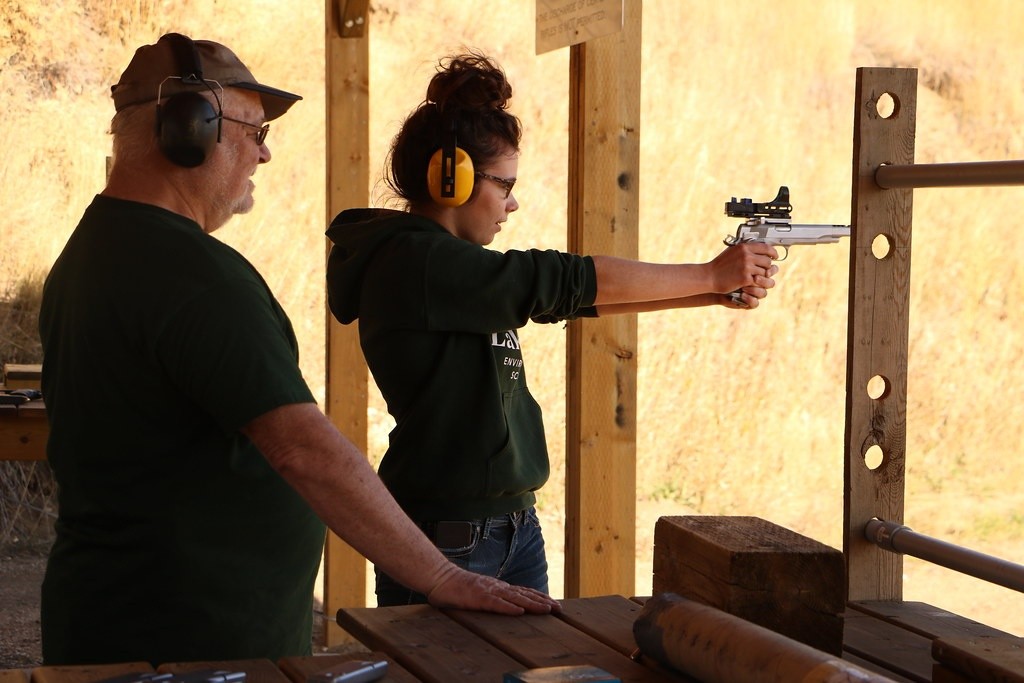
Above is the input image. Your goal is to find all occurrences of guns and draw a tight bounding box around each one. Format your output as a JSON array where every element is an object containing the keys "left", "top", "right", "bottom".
[{"left": 722, "top": 184, "right": 851, "bottom": 310}]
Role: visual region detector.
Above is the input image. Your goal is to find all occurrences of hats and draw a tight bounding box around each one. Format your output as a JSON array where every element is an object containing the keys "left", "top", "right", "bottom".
[{"left": 112, "top": 39, "right": 303, "bottom": 123}]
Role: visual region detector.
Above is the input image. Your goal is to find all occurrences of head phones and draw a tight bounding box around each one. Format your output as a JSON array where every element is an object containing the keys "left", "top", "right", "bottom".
[
  {"left": 426, "top": 68, "right": 474, "bottom": 208},
  {"left": 157, "top": 32, "right": 223, "bottom": 167}
]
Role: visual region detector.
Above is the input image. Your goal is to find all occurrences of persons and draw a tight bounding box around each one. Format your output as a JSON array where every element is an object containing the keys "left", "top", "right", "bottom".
[
  {"left": 37, "top": 32, "right": 560, "bottom": 664},
  {"left": 326, "top": 49, "right": 780, "bottom": 607}
]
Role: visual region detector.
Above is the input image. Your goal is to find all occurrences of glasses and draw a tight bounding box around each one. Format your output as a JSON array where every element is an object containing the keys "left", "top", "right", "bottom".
[
  {"left": 204, "top": 114, "right": 270, "bottom": 146},
  {"left": 472, "top": 167, "right": 516, "bottom": 198}
]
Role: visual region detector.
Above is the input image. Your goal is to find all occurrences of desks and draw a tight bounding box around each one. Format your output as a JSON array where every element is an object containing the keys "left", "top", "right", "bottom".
[{"left": 0, "top": 596, "right": 1024, "bottom": 682}]
